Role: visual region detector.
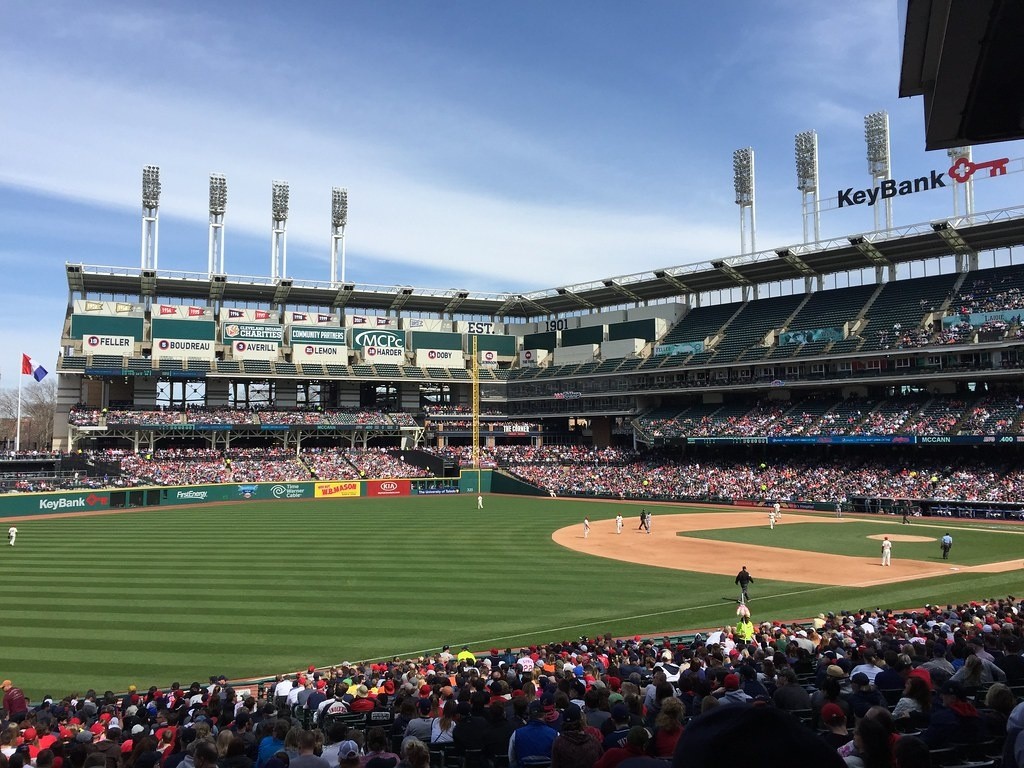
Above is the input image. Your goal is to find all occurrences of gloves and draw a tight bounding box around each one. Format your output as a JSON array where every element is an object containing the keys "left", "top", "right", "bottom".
[
  {"left": 735, "top": 580, "right": 738, "bottom": 585},
  {"left": 751, "top": 580, "right": 754, "bottom": 583}
]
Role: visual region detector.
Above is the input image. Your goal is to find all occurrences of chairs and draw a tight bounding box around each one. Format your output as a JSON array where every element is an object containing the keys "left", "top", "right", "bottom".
[{"left": 256, "top": 623, "right": 1024, "bottom": 768}]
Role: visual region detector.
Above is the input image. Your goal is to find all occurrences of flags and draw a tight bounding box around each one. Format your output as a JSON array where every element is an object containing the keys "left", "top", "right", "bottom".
[{"left": 22, "top": 354, "right": 48, "bottom": 382}]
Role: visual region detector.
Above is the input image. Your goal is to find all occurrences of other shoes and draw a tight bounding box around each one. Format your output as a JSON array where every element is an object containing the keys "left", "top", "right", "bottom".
[
  {"left": 639, "top": 527, "right": 642, "bottom": 530},
  {"left": 943, "top": 557, "right": 948, "bottom": 559}
]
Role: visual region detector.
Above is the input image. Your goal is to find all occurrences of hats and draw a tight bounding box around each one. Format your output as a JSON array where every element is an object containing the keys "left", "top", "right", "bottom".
[
  {"left": 298, "top": 678, "right": 306, "bottom": 685},
  {"left": 385, "top": 680, "right": 395, "bottom": 695},
  {"left": 357, "top": 685, "right": 369, "bottom": 698},
  {"left": 677, "top": 645, "right": 685, "bottom": 651},
  {"left": 821, "top": 703, "right": 844, "bottom": 722},
  {"left": 129, "top": 685, "right": 137, "bottom": 692},
  {"left": 562, "top": 702, "right": 582, "bottom": 722},
  {"left": 337, "top": 739, "right": 359, "bottom": 760},
  {"left": 489, "top": 648, "right": 498, "bottom": 654},
  {"left": 316, "top": 680, "right": 326, "bottom": 689},
  {"left": 24, "top": 728, "right": 36, "bottom": 740},
  {"left": 935, "top": 679, "right": 962, "bottom": 695},
  {"left": 886, "top": 626, "right": 896, "bottom": 633},
  {"left": 308, "top": 666, "right": 315, "bottom": 672},
  {"left": 332, "top": 595, "right": 1024, "bottom": 737},
  {"left": 529, "top": 699, "right": 545, "bottom": 718},
  {"left": 723, "top": 673, "right": 739, "bottom": 690},
  {"left": 796, "top": 630, "right": 807, "bottom": 637},
  {"left": 131, "top": 724, "right": 144, "bottom": 735},
  {"left": 0, "top": 680, "right": 11, "bottom": 688},
  {"left": 418, "top": 685, "right": 431, "bottom": 698},
  {"left": 59, "top": 713, "right": 120, "bottom": 742}
]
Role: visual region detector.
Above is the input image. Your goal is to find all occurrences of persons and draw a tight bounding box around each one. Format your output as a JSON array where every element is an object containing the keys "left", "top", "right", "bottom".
[
  {"left": 773, "top": 501, "right": 781, "bottom": 518},
  {"left": 615, "top": 512, "right": 624, "bottom": 533},
  {"left": 768, "top": 511, "right": 776, "bottom": 530},
  {"left": 510, "top": 447, "right": 1024, "bottom": 517},
  {"left": 639, "top": 509, "right": 651, "bottom": 534},
  {"left": 835, "top": 502, "right": 842, "bottom": 518},
  {"left": 68, "top": 400, "right": 416, "bottom": 428},
  {"left": 902, "top": 505, "right": 912, "bottom": 524},
  {"left": 640, "top": 388, "right": 1024, "bottom": 437},
  {"left": 878, "top": 273, "right": 1024, "bottom": 349},
  {"left": 941, "top": 532, "right": 952, "bottom": 559},
  {"left": 584, "top": 516, "right": 590, "bottom": 539},
  {"left": 7, "top": 524, "right": 18, "bottom": 546},
  {"left": 477, "top": 495, "right": 483, "bottom": 510},
  {"left": 736, "top": 565, "right": 755, "bottom": 603},
  {"left": 389, "top": 404, "right": 642, "bottom": 469},
  {"left": 879, "top": 536, "right": 891, "bottom": 566},
  {"left": 1, "top": 589, "right": 1024, "bottom": 768},
  {"left": 0, "top": 443, "right": 439, "bottom": 495}
]
